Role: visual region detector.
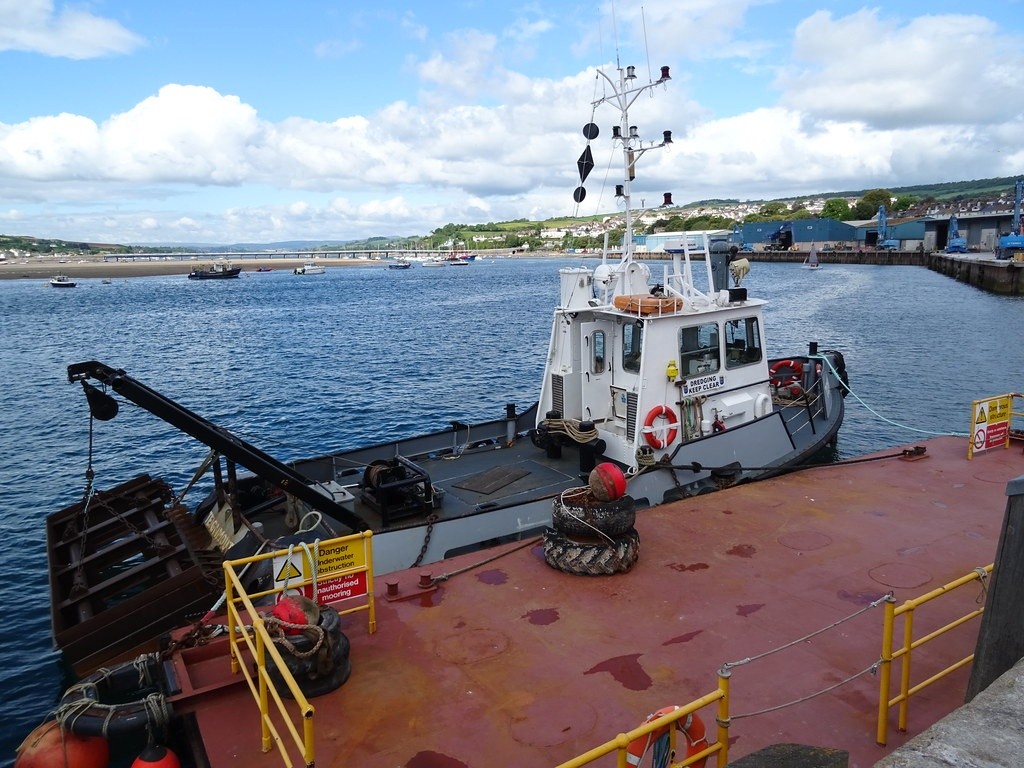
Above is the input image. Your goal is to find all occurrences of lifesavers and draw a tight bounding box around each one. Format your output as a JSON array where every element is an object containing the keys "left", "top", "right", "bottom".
[
  {"left": 769, "top": 361, "right": 803, "bottom": 387},
  {"left": 645, "top": 406, "right": 678, "bottom": 449}
]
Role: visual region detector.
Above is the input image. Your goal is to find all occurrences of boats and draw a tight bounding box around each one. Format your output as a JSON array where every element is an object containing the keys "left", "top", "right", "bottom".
[
  {"left": 449, "top": 261, "right": 469, "bottom": 265},
  {"left": 293, "top": 263, "right": 326, "bottom": 274},
  {"left": 663, "top": 240, "right": 697, "bottom": 254},
  {"left": 115, "top": 253, "right": 231, "bottom": 260},
  {"left": 50, "top": 275, "right": 77, "bottom": 287},
  {"left": 256, "top": 264, "right": 272, "bottom": 272},
  {"left": 389, "top": 258, "right": 411, "bottom": 268},
  {"left": 100, "top": 279, "right": 112, "bottom": 283},
  {"left": 68, "top": 2, "right": 849, "bottom": 605},
  {"left": 187, "top": 260, "right": 242, "bottom": 279},
  {"left": 421, "top": 259, "right": 445, "bottom": 267}
]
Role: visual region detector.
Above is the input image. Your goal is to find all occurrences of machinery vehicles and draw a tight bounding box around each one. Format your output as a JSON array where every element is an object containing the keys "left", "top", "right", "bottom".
[
  {"left": 763, "top": 220, "right": 793, "bottom": 252},
  {"left": 833, "top": 240, "right": 846, "bottom": 251},
  {"left": 732, "top": 225, "right": 753, "bottom": 250},
  {"left": 822, "top": 243, "right": 831, "bottom": 251},
  {"left": 994, "top": 177, "right": 1024, "bottom": 259},
  {"left": 875, "top": 205, "right": 898, "bottom": 251},
  {"left": 946, "top": 212, "right": 970, "bottom": 253}
]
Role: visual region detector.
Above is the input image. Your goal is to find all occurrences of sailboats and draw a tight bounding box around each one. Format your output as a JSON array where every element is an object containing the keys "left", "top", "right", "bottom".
[
  {"left": 338, "top": 239, "right": 479, "bottom": 263},
  {"left": 801, "top": 237, "right": 824, "bottom": 269}
]
